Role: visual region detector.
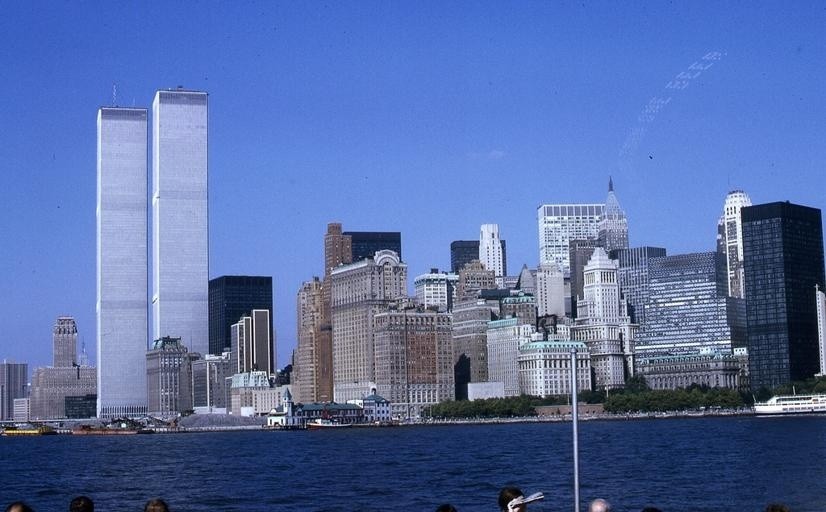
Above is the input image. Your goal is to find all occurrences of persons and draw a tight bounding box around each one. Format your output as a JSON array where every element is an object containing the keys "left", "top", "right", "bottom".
[
  {"left": 765, "top": 502, "right": 791, "bottom": 512},
  {"left": 141, "top": 498, "right": 170, "bottom": 512},
  {"left": 435, "top": 504, "right": 458, "bottom": 512},
  {"left": 67, "top": 497, "right": 96, "bottom": 512},
  {"left": 498, "top": 487, "right": 526, "bottom": 512},
  {"left": 4, "top": 502, "right": 35, "bottom": 512},
  {"left": 641, "top": 507, "right": 663, "bottom": 512},
  {"left": 588, "top": 498, "right": 612, "bottom": 512}
]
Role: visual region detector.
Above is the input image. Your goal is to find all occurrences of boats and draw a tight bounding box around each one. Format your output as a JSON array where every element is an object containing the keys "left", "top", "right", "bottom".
[
  {"left": 1, "top": 420, "right": 143, "bottom": 436},
  {"left": 753, "top": 394, "right": 826, "bottom": 415}
]
